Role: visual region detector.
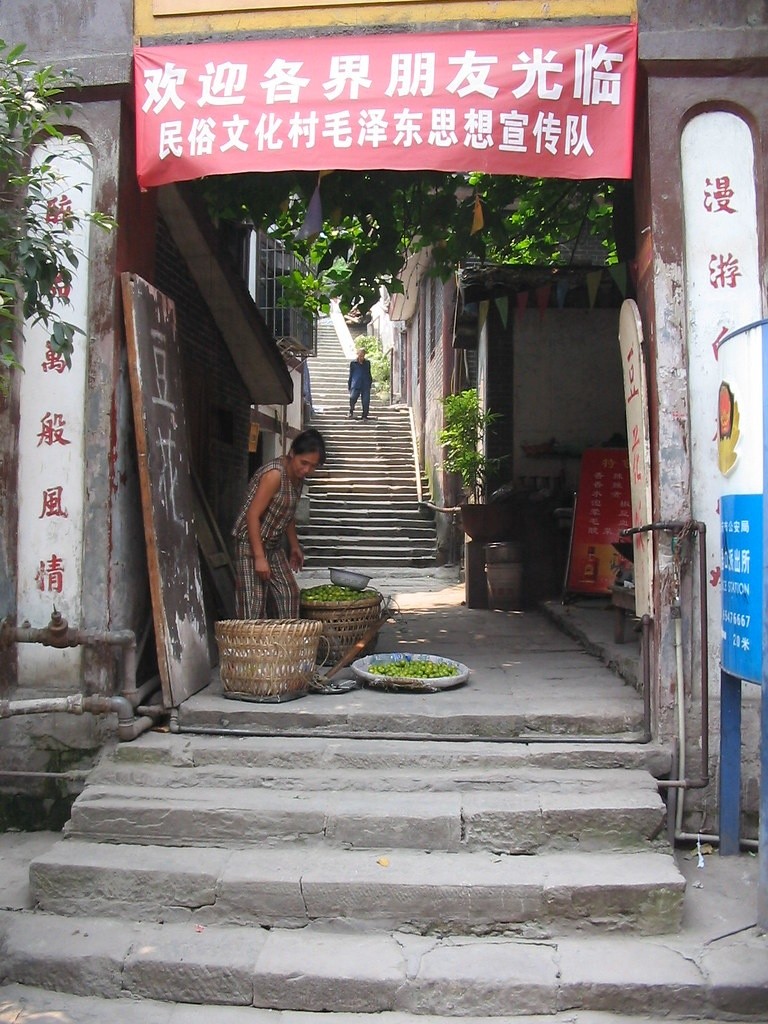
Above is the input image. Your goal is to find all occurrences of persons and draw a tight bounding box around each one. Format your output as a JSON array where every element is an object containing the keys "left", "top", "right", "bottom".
[
  {"left": 231, "top": 429, "right": 326, "bottom": 619},
  {"left": 348, "top": 348, "right": 373, "bottom": 419}
]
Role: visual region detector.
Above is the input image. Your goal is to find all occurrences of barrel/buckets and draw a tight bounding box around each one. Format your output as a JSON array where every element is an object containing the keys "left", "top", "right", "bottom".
[{"left": 483, "top": 562, "right": 524, "bottom": 611}]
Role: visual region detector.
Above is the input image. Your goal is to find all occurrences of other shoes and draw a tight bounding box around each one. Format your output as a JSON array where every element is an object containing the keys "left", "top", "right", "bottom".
[
  {"left": 349, "top": 410, "right": 353, "bottom": 416},
  {"left": 362, "top": 415, "right": 368, "bottom": 420}
]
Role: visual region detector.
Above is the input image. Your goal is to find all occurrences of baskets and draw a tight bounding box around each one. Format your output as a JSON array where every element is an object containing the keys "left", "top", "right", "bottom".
[
  {"left": 300, "top": 594, "right": 384, "bottom": 667},
  {"left": 215, "top": 618, "right": 323, "bottom": 703}
]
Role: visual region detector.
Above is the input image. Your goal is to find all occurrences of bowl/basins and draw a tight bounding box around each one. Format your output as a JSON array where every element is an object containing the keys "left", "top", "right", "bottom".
[
  {"left": 521, "top": 437, "right": 555, "bottom": 456},
  {"left": 328, "top": 565, "right": 373, "bottom": 591}
]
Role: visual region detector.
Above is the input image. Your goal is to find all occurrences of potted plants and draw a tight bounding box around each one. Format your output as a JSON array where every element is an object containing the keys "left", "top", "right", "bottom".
[{"left": 441, "top": 388, "right": 513, "bottom": 540}]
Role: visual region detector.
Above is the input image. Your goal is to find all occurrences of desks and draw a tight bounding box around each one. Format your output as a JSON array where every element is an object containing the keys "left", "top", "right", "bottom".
[{"left": 607, "top": 584, "right": 641, "bottom": 643}]
